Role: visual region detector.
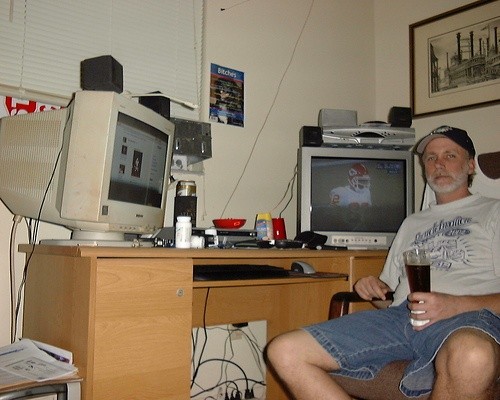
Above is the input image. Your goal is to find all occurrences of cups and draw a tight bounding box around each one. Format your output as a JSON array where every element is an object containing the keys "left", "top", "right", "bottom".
[
  {"left": 405, "top": 248, "right": 431, "bottom": 327},
  {"left": 272, "top": 218, "right": 286, "bottom": 240},
  {"left": 174, "top": 196, "right": 197, "bottom": 228}
]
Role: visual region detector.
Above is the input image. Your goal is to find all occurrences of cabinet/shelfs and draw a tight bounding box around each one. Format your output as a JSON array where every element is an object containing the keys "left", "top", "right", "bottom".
[{"left": 18, "top": 243, "right": 401, "bottom": 400}]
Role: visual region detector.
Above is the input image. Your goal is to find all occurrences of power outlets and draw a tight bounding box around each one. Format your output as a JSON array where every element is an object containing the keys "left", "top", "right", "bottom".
[{"left": 227, "top": 323, "right": 243, "bottom": 340}]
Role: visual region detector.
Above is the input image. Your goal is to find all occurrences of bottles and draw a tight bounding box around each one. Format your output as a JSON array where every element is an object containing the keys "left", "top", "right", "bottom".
[{"left": 176, "top": 216, "right": 192, "bottom": 248}]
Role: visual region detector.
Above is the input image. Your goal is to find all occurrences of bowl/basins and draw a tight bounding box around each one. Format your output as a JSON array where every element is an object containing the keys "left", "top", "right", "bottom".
[{"left": 213, "top": 218, "right": 246, "bottom": 229}]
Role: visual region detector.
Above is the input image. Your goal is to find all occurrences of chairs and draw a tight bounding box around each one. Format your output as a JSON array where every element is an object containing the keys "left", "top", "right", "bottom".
[{"left": 326, "top": 150, "right": 500, "bottom": 400}]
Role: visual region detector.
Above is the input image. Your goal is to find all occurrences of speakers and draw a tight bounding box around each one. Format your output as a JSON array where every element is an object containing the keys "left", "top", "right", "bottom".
[
  {"left": 79, "top": 55, "right": 123, "bottom": 93},
  {"left": 388, "top": 107, "right": 413, "bottom": 128},
  {"left": 139, "top": 92, "right": 171, "bottom": 120},
  {"left": 299, "top": 126, "right": 322, "bottom": 147}
]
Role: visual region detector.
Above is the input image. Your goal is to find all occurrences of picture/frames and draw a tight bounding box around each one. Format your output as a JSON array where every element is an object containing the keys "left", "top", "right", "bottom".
[{"left": 408, "top": 0, "right": 500, "bottom": 119}]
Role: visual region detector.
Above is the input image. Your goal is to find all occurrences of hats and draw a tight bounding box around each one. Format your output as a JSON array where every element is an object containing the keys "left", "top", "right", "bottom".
[{"left": 416, "top": 125, "right": 475, "bottom": 159}]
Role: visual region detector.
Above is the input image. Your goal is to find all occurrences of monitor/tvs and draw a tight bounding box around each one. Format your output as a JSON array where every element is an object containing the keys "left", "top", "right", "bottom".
[
  {"left": 297, "top": 147, "right": 414, "bottom": 251},
  {"left": 0, "top": 90, "right": 175, "bottom": 247}
]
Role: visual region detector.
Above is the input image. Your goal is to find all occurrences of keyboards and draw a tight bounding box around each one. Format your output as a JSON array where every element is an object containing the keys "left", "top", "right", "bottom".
[{"left": 193, "top": 264, "right": 289, "bottom": 282}]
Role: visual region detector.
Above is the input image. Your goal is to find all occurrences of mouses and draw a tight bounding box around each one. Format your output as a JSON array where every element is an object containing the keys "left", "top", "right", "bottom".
[{"left": 291, "top": 261, "right": 316, "bottom": 274}]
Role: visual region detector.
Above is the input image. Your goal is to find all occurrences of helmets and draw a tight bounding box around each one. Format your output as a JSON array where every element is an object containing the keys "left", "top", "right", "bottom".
[{"left": 348, "top": 163, "right": 371, "bottom": 192}]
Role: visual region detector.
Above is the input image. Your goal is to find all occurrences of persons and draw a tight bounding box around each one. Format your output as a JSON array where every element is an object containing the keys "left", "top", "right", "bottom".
[
  {"left": 329, "top": 163, "right": 373, "bottom": 231},
  {"left": 266, "top": 126, "right": 500, "bottom": 400}
]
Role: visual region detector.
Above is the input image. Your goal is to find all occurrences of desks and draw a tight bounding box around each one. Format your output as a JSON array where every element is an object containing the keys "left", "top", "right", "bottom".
[{"left": 0, "top": 344, "right": 84, "bottom": 400}]
